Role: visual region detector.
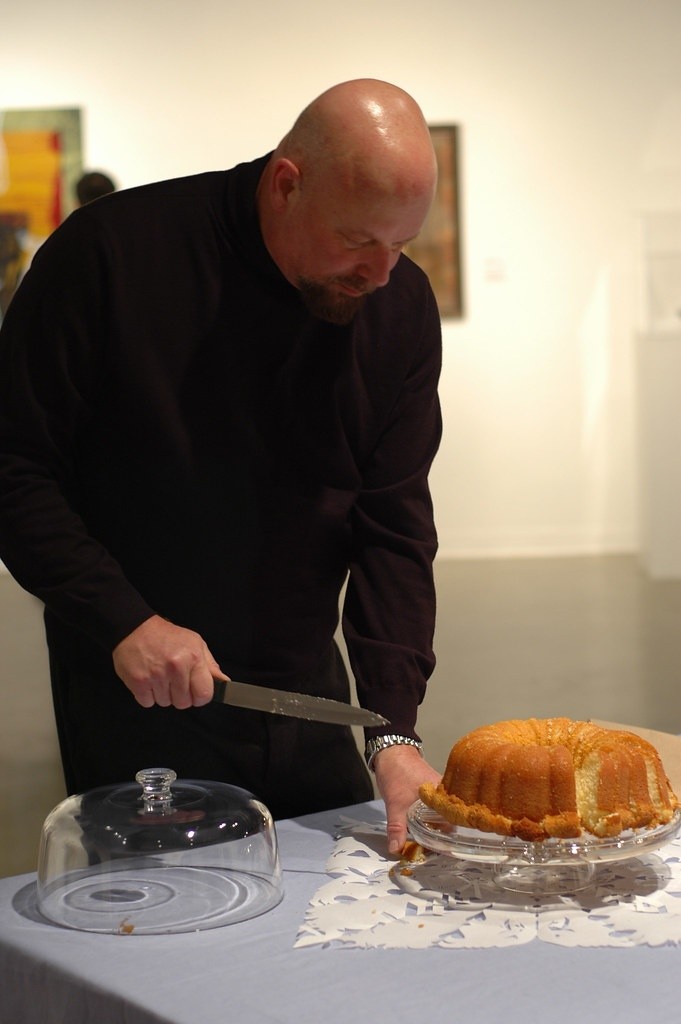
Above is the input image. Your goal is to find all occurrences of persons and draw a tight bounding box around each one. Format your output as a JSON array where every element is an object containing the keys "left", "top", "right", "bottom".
[
  {"left": 75, "top": 171, "right": 114, "bottom": 206},
  {"left": 0, "top": 224, "right": 33, "bottom": 313},
  {"left": 0, "top": 80, "right": 446, "bottom": 868}
]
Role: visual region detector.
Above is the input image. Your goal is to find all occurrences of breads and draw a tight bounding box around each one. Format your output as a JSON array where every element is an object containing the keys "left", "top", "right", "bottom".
[
  {"left": 415, "top": 718, "right": 681, "bottom": 841},
  {"left": 399, "top": 839, "right": 432, "bottom": 861}
]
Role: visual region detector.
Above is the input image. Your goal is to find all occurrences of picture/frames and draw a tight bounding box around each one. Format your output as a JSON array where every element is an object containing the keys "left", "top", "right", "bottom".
[
  {"left": 401, "top": 123, "right": 464, "bottom": 319},
  {"left": 0, "top": 108, "right": 82, "bottom": 272}
]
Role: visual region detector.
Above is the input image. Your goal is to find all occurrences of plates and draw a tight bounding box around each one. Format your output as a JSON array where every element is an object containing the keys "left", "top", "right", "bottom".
[{"left": 405, "top": 797, "right": 681, "bottom": 896}]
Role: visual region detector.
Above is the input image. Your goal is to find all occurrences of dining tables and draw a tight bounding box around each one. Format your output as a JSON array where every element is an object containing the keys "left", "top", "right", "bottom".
[{"left": 0, "top": 797, "right": 681, "bottom": 1024}]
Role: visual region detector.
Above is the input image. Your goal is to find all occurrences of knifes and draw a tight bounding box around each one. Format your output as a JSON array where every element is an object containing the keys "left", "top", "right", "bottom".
[{"left": 210, "top": 676, "right": 392, "bottom": 727}]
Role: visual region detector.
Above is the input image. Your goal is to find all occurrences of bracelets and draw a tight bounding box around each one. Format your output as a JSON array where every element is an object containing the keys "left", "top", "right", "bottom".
[{"left": 364, "top": 736, "right": 425, "bottom": 775}]
